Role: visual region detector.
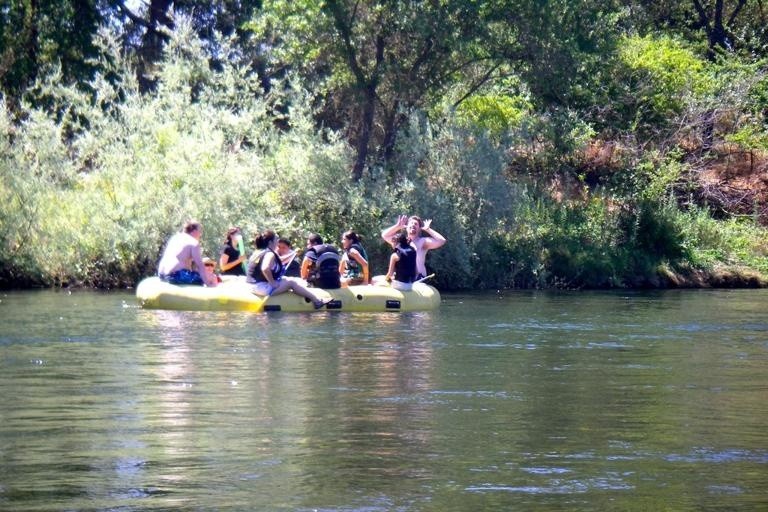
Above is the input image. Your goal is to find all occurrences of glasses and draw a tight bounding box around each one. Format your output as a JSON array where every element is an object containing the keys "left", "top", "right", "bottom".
[{"left": 205, "top": 264, "right": 215, "bottom": 266}]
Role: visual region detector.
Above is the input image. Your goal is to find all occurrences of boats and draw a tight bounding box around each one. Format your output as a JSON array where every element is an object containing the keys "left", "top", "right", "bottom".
[{"left": 136, "top": 275, "right": 442, "bottom": 312}]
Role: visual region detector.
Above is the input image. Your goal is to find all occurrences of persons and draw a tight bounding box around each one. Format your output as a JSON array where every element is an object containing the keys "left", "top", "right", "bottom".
[
  {"left": 300, "top": 233, "right": 355, "bottom": 288},
  {"left": 385, "top": 232, "right": 417, "bottom": 293},
  {"left": 155, "top": 221, "right": 217, "bottom": 287},
  {"left": 246, "top": 230, "right": 335, "bottom": 308},
  {"left": 381, "top": 214, "right": 446, "bottom": 279},
  {"left": 201, "top": 255, "right": 223, "bottom": 283},
  {"left": 220, "top": 226, "right": 248, "bottom": 275},
  {"left": 339, "top": 230, "right": 372, "bottom": 286},
  {"left": 274, "top": 236, "right": 300, "bottom": 278}
]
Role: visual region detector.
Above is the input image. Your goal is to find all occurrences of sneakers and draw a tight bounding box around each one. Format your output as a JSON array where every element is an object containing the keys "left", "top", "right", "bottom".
[{"left": 314, "top": 297, "right": 334, "bottom": 308}]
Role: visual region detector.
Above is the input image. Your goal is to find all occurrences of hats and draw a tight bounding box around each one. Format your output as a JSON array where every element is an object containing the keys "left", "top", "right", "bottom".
[{"left": 201, "top": 257, "right": 217, "bottom": 265}]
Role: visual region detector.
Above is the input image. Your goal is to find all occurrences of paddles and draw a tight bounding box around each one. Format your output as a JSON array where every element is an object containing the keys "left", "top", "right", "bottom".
[{"left": 249, "top": 248, "right": 298, "bottom": 313}]
